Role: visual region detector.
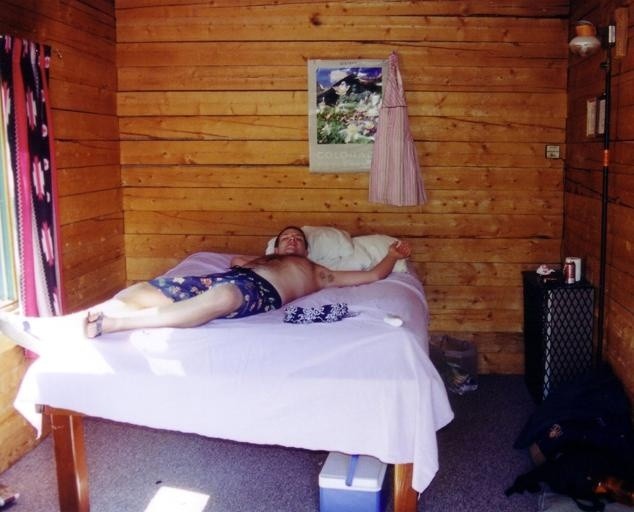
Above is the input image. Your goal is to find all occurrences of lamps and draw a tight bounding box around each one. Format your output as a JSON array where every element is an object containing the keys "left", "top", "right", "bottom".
[{"left": 568, "top": 20, "right": 601, "bottom": 59}]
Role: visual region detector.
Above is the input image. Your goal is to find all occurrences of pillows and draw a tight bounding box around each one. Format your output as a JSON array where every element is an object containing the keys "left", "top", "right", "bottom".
[{"left": 266, "top": 225, "right": 408, "bottom": 273}]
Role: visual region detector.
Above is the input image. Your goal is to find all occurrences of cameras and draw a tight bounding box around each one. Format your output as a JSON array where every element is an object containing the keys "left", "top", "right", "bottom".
[{"left": 537, "top": 273, "right": 559, "bottom": 285}]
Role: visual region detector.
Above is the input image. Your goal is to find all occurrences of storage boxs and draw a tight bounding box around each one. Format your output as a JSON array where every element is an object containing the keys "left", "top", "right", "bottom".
[{"left": 318, "top": 452, "right": 388, "bottom": 512}]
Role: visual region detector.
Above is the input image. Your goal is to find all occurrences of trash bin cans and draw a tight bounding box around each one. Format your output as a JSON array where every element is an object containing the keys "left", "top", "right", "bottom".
[{"left": 429, "top": 336, "right": 477, "bottom": 394}]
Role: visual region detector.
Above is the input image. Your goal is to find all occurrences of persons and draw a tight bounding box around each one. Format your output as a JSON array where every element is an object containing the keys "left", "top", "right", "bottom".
[{"left": 86, "top": 226, "right": 414, "bottom": 338}]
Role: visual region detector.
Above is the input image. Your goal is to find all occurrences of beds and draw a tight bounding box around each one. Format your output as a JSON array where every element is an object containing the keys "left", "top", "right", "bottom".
[{"left": 12, "top": 252, "right": 454, "bottom": 512}]
